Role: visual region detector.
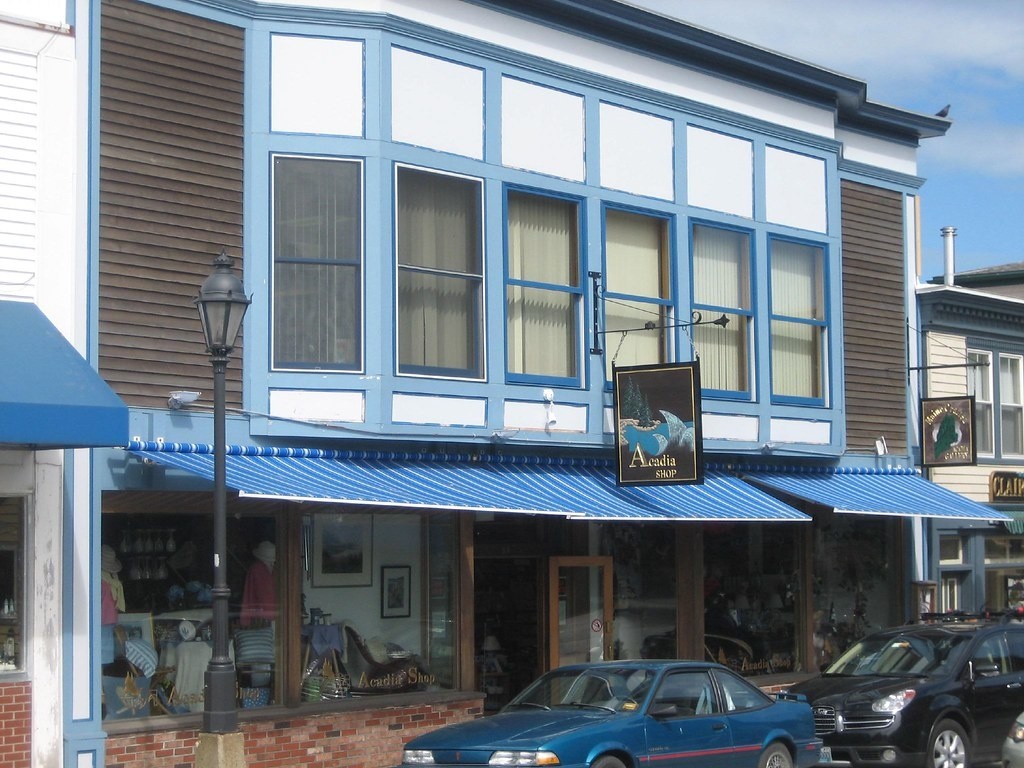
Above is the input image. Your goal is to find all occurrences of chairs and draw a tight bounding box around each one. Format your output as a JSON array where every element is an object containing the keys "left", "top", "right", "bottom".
[
  {"left": 114, "top": 624, "right": 177, "bottom": 715},
  {"left": 662, "top": 688, "right": 692, "bottom": 716}
]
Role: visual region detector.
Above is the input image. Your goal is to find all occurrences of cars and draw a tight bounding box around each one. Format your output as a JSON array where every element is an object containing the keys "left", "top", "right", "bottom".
[{"left": 393, "top": 658, "right": 825, "bottom": 768}]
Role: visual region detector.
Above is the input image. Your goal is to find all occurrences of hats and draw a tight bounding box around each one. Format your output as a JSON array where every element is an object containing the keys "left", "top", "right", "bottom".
[
  {"left": 251, "top": 541, "right": 276, "bottom": 564},
  {"left": 101, "top": 544, "right": 122, "bottom": 573}
]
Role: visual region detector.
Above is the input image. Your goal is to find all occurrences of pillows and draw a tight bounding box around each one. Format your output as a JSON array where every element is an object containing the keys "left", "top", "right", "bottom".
[
  {"left": 125, "top": 637, "right": 159, "bottom": 679},
  {"left": 234, "top": 627, "right": 275, "bottom": 665},
  {"left": 102, "top": 676, "right": 151, "bottom": 720}
]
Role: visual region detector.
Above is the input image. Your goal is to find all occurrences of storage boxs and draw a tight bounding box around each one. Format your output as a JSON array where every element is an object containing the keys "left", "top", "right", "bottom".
[{"left": 240, "top": 672, "right": 271, "bottom": 708}]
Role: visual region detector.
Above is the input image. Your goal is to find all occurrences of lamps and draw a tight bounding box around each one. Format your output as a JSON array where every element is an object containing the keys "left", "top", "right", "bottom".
[
  {"left": 481, "top": 636, "right": 501, "bottom": 657},
  {"left": 167, "top": 391, "right": 202, "bottom": 410},
  {"left": 543, "top": 389, "right": 558, "bottom": 428}
]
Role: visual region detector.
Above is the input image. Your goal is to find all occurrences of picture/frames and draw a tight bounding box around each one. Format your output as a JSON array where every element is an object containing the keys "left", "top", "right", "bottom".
[
  {"left": 311, "top": 510, "right": 374, "bottom": 588},
  {"left": 119, "top": 612, "right": 156, "bottom": 651},
  {"left": 381, "top": 566, "right": 412, "bottom": 618}
]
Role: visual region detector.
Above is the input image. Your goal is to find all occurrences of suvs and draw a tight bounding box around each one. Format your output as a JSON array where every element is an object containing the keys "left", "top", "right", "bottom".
[{"left": 780, "top": 609, "right": 1024, "bottom": 768}]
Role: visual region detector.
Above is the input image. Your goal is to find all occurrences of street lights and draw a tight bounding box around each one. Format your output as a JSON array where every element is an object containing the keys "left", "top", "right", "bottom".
[{"left": 191, "top": 247, "right": 256, "bottom": 735}]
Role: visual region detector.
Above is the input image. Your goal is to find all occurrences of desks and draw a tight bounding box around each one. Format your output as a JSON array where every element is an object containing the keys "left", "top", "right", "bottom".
[{"left": 157, "top": 640, "right": 238, "bottom": 711}]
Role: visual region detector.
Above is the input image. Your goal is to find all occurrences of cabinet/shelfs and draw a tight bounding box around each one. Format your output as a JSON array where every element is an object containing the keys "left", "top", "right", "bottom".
[{"left": 481, "top": 672, "right": 513, "bottom": 710}]
